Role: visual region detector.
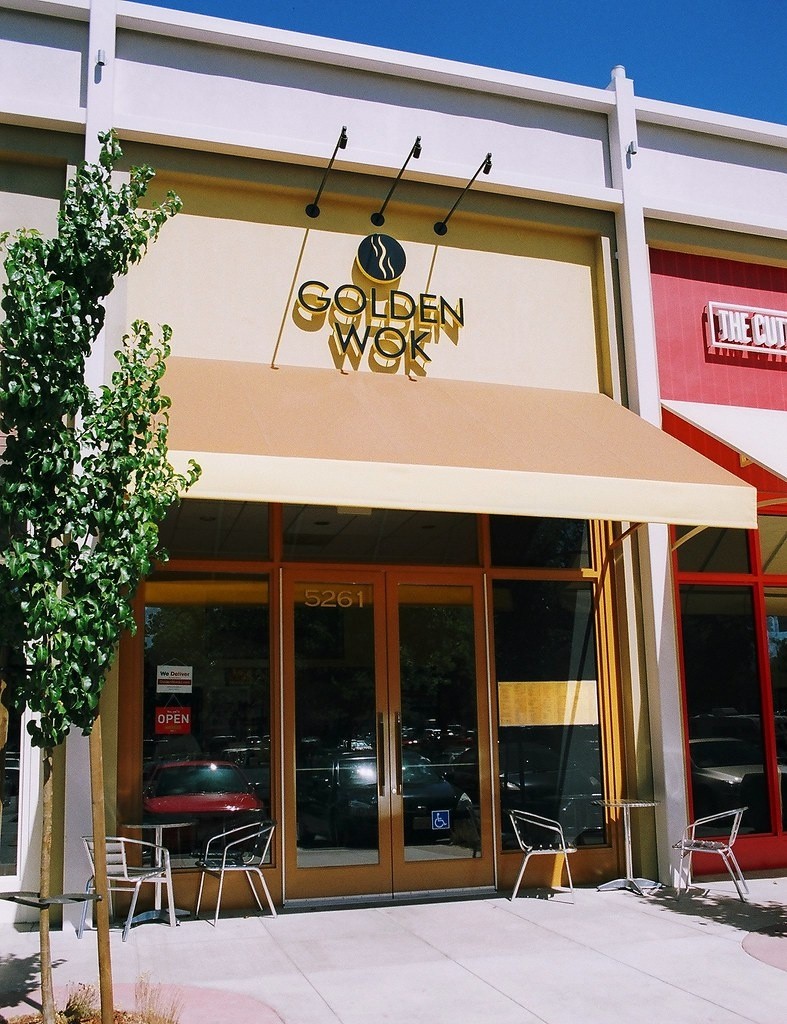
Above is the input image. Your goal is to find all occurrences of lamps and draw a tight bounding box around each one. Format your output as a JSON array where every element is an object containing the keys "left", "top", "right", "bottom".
[
  {"left": 371, "top": 136, "right": 422, "bottom": 226},
  {"left": 305, "top": 126, "right": 349, "bottom": 219},
  {"left": 433, "top": 153, "right": 493, "bottom": 236}
]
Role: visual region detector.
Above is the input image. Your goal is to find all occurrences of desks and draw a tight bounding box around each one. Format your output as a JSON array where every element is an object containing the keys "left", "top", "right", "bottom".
[
  {"left": 121, "top": 821, "right": 196, "bottom": 926},
  {"left": 589, "top": 799, "right": 666, "bottom": 897}
]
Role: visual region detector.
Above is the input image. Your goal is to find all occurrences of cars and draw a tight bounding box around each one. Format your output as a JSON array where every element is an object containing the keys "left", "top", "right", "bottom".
[
  {"left": 691, "top": 763, "right": 787, "bottom": 824},
  {"left": 338, "top": 739, "right": 371, "bottom": 752},
  {"left": 248, "top": 736, "right": 261, "bottom": 746},
  {"left": 141, "top": 761, "right": 262, "bottom": 851},
  {"left": 449, "top": 747, "right": 587, "bottom": 841},
  {"left": 300, "top": 754, "right": 450, "bottom": 841},
  {"left": 206, "top": 736, "right": 237, "bottom": 748},
  {"left": 376, "top": 716, "right": 482, "bottom": 760},
  {"left": 689, "top": 705, "right": 787, "bottom": 766}
]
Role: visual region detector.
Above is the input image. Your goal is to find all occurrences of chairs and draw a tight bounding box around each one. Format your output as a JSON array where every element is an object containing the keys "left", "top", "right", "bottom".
[
  {"left": 195, "top": 819, "right": 278, "bottom": 927},
  {"left": 508, "top": 810, "right": 578, "bottom": 900},
  {"left": 78, "top": 835, "right": 180, "bottom": 942},
  {"left": 671, "top": 807, "right": 749, "bottom": 902}
]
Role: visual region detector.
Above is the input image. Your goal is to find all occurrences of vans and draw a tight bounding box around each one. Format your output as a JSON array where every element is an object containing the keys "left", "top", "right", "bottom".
[{"left": 218, "top": 748, "right": 264, "bottom": 766}]
